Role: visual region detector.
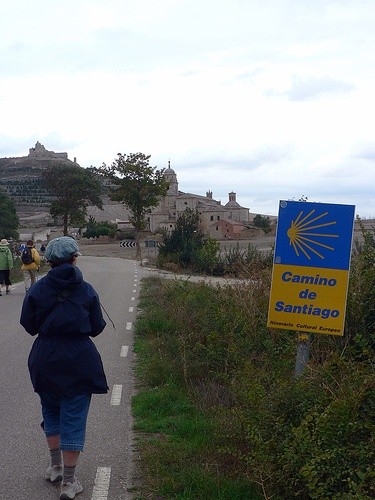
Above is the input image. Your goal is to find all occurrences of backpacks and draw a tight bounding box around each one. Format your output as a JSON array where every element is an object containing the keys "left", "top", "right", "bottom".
[{"left": 22, "top": 247, "right": 34, "bottom": 264}]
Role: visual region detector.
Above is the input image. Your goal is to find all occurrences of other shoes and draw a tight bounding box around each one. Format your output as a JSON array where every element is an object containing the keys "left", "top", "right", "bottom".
[
  {"left": 0, "top": 291, "right": 3, "bottom": 296},
  {"left": 6, "top": 289, "right": 11, "bottom": 295}
]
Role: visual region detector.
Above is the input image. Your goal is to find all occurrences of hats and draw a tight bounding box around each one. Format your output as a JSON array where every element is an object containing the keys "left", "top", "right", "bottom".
[
  {"left": 45, "top": 236, "right": 82, "bottom": 262},
  {"left": 0, "top": 239, "right": 9, "bottom": 246}
]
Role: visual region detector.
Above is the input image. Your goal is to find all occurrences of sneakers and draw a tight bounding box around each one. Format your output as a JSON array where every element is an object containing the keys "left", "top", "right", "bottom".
[
  {"left": 59, "top": 475, "right": 83, "bottom": 500},
  {"left": 45, "top": 465, "right": 63, "bottom": 482}
]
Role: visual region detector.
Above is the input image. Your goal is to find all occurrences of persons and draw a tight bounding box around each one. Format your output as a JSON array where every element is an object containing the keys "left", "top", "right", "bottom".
[
  {"left": 19, "top": 236, "right": 109, "bottom": 499},
  {"left": 0, "top": 238, "right": 13, "bottom": 296},
  {"left": 21, "top": 240, "right": 41, "bottom": 294}
]
